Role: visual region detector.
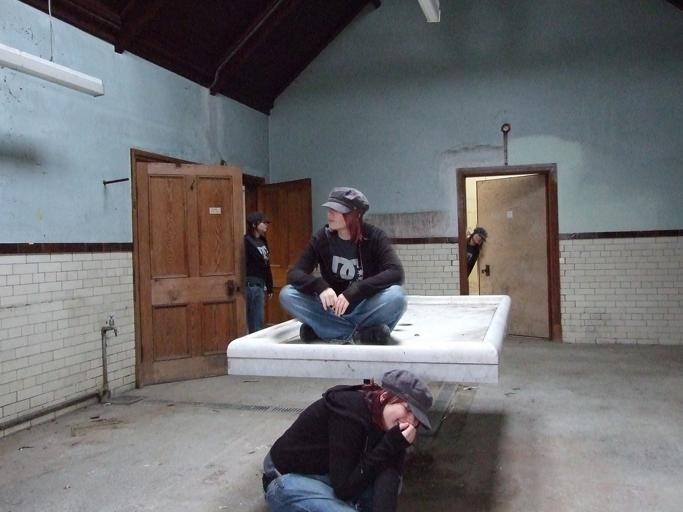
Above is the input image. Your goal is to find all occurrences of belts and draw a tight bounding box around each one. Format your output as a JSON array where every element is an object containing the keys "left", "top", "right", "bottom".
[{"left": 247, "top": 282, "right": 262, "bottom": 287}]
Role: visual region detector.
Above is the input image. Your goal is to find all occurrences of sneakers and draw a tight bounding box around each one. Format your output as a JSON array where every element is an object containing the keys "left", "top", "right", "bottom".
[
  {"left": 299, "top": 324, "right": 316, "bottom": 343},
  {"left": 360, "top": 324, "right": 389, "bottom": 345}
]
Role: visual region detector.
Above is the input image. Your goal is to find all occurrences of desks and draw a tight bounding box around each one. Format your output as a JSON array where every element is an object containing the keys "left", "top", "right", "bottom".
[{"left": 223, "top": 287, "right": 512, "bottom": 476}]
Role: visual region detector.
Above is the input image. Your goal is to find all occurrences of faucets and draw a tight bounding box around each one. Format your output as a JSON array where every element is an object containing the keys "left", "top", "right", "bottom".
[{"left": 103, "top": 314, "right": 118, "bottom": 337}]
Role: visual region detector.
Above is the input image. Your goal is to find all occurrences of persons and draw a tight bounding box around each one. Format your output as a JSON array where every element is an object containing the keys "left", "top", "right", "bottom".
[
  {"left": 277, "top": 185, "right": 407, "bottom": 347},
  {"left": 463, "top": 227, "right": 488, "bottom": 277},
  {"left": 242, "top": 213, "right": 273, "bottom": 336},
  {"left": 259, "top": 367, "right": 433, "bottom": 511}
]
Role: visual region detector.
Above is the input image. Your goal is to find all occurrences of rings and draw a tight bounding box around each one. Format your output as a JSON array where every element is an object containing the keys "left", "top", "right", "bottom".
[{"left": 329, "top": 305, "right": 333, "bottom": 308}]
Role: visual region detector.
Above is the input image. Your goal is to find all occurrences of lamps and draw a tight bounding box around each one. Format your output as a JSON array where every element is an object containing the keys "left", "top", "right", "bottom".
[
  {"left": 416, "top": 1, "right": 442, "bottom": 25},
  {"left": 0, "top": 0, "right": 105, "bottom": 99}
]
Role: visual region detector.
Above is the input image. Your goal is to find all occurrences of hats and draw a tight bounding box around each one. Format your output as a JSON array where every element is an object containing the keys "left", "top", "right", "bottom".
[
  {"left": 474, "top": 228, "right": 487, "bottom": 240},
  {"left": 246, "top": 211, "right": 271, "bottom": 223},
  {"left": 321, "top": 186, "right": 369, "bottom": 214},
  {"left": 381, "top": 368, "right": 434, "bottom": 431}
]
